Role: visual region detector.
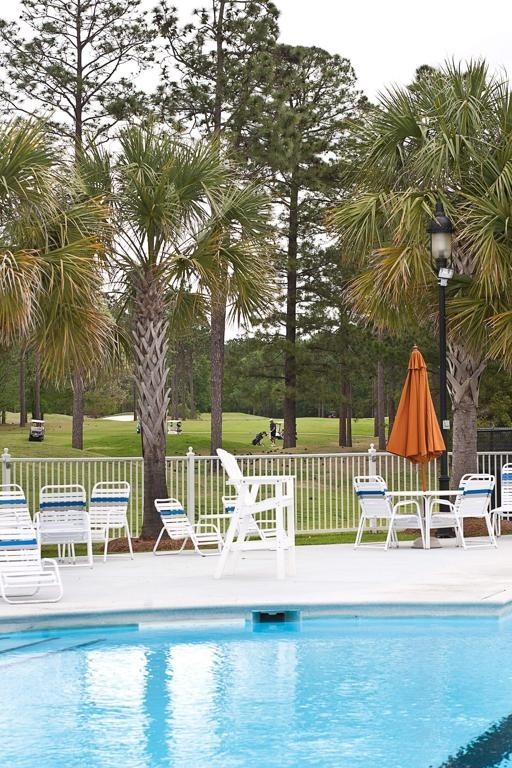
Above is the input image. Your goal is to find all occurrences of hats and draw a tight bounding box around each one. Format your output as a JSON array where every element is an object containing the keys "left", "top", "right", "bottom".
[{"left": 268, "top": 419, "right": 273, "bottom": 422}]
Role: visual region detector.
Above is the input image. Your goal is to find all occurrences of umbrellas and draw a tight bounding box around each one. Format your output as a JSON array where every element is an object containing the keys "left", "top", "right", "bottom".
[{"left": 385, "top": 345, "right": 447, "bottom": 491}]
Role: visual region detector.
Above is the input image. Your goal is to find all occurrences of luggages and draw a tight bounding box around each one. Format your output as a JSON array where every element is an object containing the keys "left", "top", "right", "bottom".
[{"left": 251, "top": 431, "right": 269, "bottom": 446}]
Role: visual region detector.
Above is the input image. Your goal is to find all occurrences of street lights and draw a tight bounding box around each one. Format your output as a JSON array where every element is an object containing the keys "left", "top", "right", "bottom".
[{"left": 424, "top": 202, "right": 465, "bottom": 539}]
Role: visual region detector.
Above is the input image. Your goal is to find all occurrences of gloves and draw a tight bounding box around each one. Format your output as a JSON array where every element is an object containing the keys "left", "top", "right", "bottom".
[{"left": 269, "top": 431, "right": 272, "bottom": 433}]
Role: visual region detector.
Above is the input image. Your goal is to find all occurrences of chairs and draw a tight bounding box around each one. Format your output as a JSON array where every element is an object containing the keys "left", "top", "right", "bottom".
[
  {"left": 215, "top": 448, "right": 296, "bottom": 581},
  {"left": 353, "top": 473, "right": 498, "bottom": 550},
  {"left": 153, "top": 498, "right": 225, "bottom": 556},
  {"left": 1, "top": 484, "right": 62, "bottom": 604},
  {"left": 89, "top": 481, "right": 134, "bottom": 563},
  {"left": 34, "top": 485, "right": 93, "bottom": 572},
  {"left": 495, "top": 463, "right": 512, "bottom": 537}
]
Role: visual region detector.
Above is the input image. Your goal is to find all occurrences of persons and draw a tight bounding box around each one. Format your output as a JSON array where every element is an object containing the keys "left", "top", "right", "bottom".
[
  {"left": 278, "top": 429, "right": 284, "bottom": 440},
  {"left": 269, "top": 419, "right": 276, "bottom": 447}
]
